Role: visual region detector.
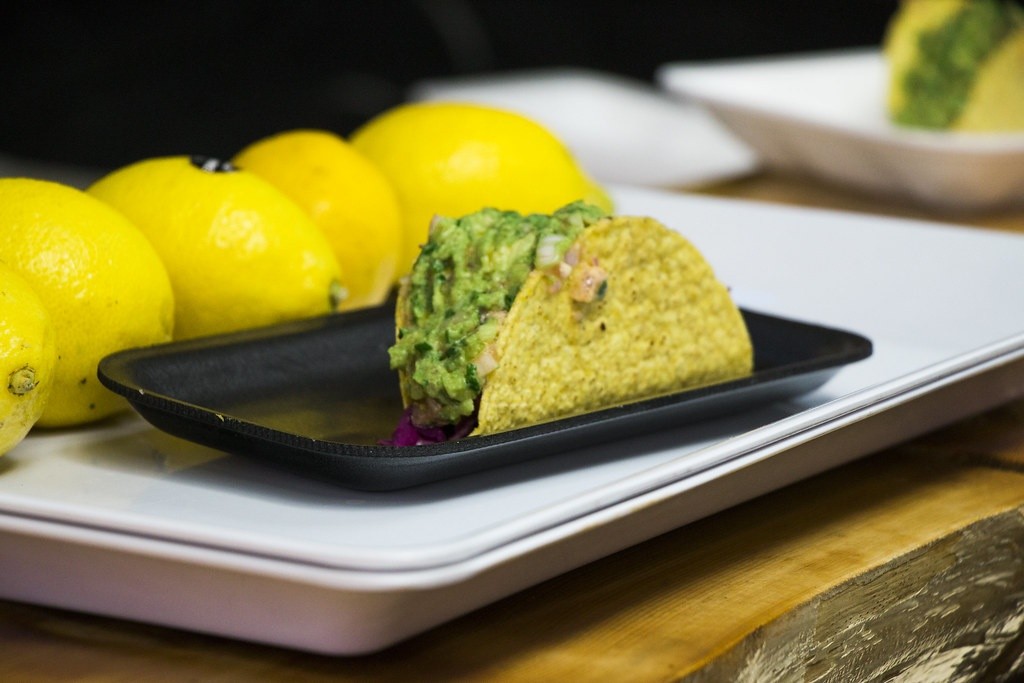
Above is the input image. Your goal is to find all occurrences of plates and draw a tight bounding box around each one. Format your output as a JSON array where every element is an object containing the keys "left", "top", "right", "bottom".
[
  {"left": 664, "top": 52, "right": 1024, "bottom": 207},
  {"left": 98, "top": 306, "right": 874, "bottom": 491}
]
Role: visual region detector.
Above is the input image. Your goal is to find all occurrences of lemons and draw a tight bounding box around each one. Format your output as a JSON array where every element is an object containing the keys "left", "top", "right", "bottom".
[{"left": 0, "top": 99, "right": 616, "bottom": 461}]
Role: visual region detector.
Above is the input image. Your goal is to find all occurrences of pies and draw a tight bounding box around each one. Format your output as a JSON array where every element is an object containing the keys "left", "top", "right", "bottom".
[
  {"left": 883, "top": 0, "right": 1024, "bottom": 133},
  {"left": 395, "top": 202, "right": 753, "bottom": 445}
]
